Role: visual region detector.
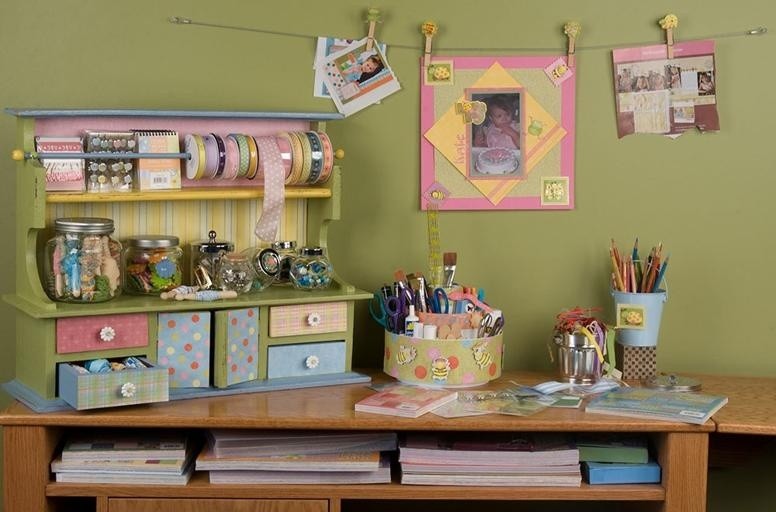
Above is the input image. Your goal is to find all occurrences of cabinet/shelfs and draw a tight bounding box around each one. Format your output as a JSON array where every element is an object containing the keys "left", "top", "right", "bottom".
[{"left": 3, "top": 108, "right": 374, "bottom": 411}]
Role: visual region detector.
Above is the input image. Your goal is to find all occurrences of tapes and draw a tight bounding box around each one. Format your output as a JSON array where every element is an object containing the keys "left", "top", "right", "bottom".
[{"left": 184, "top": 130, "right": 333, "bottom": 242}]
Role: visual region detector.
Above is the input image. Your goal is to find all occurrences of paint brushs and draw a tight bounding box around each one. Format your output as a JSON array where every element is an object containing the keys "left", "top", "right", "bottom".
[{"left": 395, "top": 253, "right": 457, "bottom": 312}]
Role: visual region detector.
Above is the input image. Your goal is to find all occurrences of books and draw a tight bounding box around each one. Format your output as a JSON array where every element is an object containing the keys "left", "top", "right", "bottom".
[
  {"left": 47, "top": 426, "right": 664, "bottom": 489},
  {"left": 355, "top": 381, "right": 458, "bottom": 419},
  {"left": 33, "top": 126, "right": 183, "bottom": 192},
  {"left": 582, "top": 383, "right": 730, "bottom": 428}
]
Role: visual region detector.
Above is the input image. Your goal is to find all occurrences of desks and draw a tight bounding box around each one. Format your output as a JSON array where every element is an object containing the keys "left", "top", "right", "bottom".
[{"left": 0, "top": 369, "right": 776, "bottom": 512}]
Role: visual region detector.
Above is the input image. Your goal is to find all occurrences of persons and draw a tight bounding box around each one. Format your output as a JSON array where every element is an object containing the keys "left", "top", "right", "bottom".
[
  {"left": 697, "top": 72, "right": 716, "bottom": 96},
  {"left": 340, "top": 54, "right": 381, "bottom": 89},
  {"left": 667, "top": 66, "right": 681, "bottom": 89},
  {"left": 617, "top": 67, "right": 633, "bottom": 92},
  {"left": 632, "top": 76, "right": 650, "bottom": 93},
  {"left": 650, "top": 73, "right": 666, "bottom": 90},
  {"left": 485, "top": 94, "right": 521, "bottom": 151}
]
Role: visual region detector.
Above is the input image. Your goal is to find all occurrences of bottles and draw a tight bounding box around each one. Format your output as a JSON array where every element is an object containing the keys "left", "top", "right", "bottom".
[
  {"left": 558, "top": 330, "right": 601, "bottom": 387},
  {"left": 123, "top": 234, "right": 185, "bottom": 298},
  {"left": 189, "top": 240, "right": 334, "bottom": 293},
  {"left": 43, "top": 216, "right": 123, "bottom": 303}
]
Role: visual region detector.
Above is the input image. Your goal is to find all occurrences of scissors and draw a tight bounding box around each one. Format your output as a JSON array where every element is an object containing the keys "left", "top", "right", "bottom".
[{"left": 369, "top": 288, "right": 449, "bottom": 334}]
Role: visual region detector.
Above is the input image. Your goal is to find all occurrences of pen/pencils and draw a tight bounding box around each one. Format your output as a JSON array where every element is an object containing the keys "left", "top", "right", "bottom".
[
  {"left": 464, "top": 287, "right": 485, "bottom": 311},
  {"left": 608, "top": 237, "right": 669, "bottom": 293}
]
